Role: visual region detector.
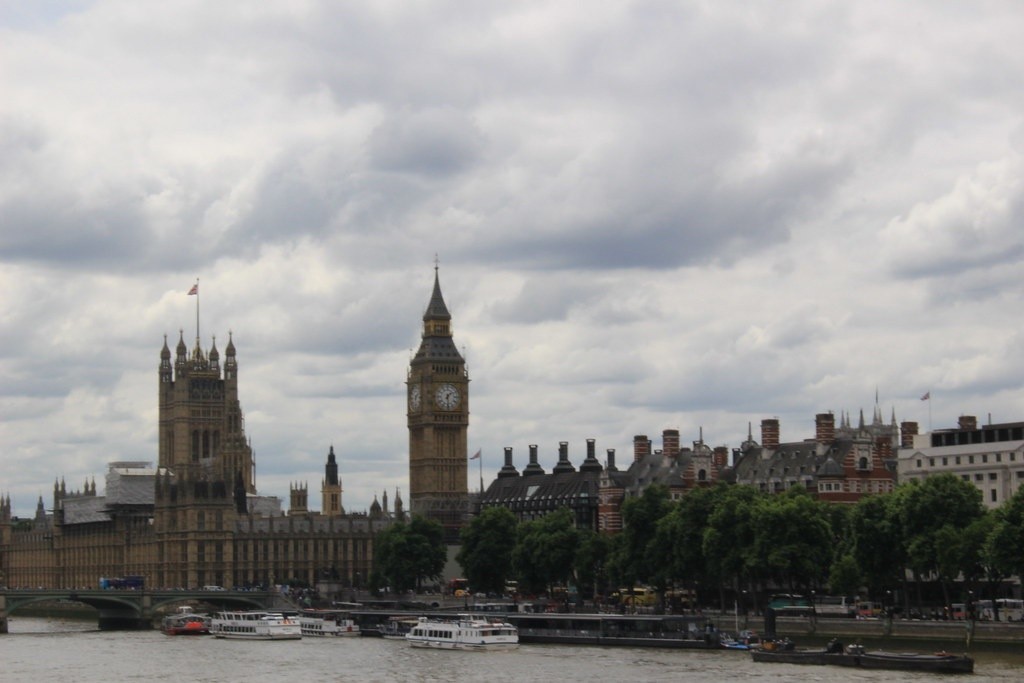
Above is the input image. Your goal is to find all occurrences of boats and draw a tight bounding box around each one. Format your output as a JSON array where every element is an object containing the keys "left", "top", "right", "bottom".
[
  {"left": 207, "top": 608, "right": 303, "bottom": 640},
  {"left": 362, "top": 615, "right": 425, "bottom": 640},
  {"left": 749, "top": 641, "right": 975, "bottom": 673},
  {"left": 162, "top": 605, "right": 211, "bottom": 636},
  {"left": 291, "top": 610, "right": 362, "bottom": 637},
  {"left": 405, "top": 614, "right": 520, "bottom": 652}
]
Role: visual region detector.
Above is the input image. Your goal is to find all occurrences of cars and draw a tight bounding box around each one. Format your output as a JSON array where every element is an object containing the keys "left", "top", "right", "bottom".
[{"left": 858, "top": 601, "right": 967, "bottom": 622}]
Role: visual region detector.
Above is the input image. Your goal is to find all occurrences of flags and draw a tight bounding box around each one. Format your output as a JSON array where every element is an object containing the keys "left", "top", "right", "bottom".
[
  {"left": 469, "top": 450, "right": 480, "bottom": 460},
  {"left": 921, "top": 392, "right": 930, "bottom": 401},
  {"left": 187, "top": 285, "right": 198, "bottom": 295}
]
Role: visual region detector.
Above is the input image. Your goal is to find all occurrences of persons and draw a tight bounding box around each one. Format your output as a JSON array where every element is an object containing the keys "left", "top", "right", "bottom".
[{"left": 703, "top": 620, "right": 714, "bottom": 641}]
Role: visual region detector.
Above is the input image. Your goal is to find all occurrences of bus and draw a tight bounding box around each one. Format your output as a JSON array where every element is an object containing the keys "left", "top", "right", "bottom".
[
  {"left": 609, "top": 586, "right": 656, "bottom": 608},
  {"left": 978, "top": 598, "right": 1024, "bottom": 623}
]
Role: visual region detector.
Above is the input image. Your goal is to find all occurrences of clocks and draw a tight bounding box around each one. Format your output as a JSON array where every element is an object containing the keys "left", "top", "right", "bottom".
[
  {"left": 435, "top": 384, "right": 461, "bottom": 411},
  {"left": 409, "top": 384, "right": 422, "bottom": 413}
]
